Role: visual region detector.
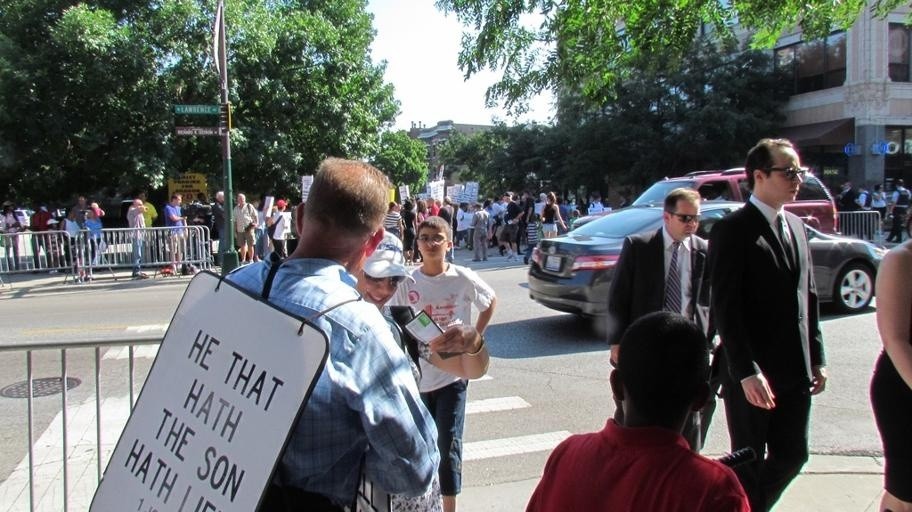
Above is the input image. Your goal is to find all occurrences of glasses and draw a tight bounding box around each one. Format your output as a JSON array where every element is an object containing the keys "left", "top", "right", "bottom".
[
  {"left": 770, "top": 168, "right": 806, "bottom": 181},
  {"left": 670, "top": 210, "right": 702, "bottom": 224},
  {"left": 362, "top": 271, "right": 400, "bottom": 285}
]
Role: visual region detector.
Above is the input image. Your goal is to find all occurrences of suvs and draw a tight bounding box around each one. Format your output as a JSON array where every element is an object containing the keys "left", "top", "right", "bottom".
[{"left": 572, "top": 166, "right": 839, "bottom": 233}]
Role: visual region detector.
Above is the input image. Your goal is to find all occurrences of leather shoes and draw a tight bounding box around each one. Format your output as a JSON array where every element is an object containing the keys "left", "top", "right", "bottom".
[{"left": 523, "top": 257, "right": 529, "bottom": 264}]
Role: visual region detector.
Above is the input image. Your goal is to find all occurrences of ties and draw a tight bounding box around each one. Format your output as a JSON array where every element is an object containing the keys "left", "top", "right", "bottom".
[
  {"left": 776, "top": 214, "right": 796, "bottom": 282},
  {"left": 665, "top": 240, "right": 682, "bottom": 317}
]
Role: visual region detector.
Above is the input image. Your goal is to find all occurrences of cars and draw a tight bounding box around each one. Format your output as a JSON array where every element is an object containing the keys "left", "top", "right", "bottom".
[{"left": 528, "top": 202, "right": 886, "bottom": 319}]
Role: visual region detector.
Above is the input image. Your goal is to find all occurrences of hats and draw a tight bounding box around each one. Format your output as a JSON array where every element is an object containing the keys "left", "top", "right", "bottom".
[
  {"left": 360, "top": 231, "right": 417, "bottom": 286},
  {"left": 503, "top": 193, "right": 512, "bottom": 197}
]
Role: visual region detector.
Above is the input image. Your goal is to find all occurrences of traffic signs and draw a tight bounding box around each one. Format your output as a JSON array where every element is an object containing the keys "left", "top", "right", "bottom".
[{"left": 173, "top": 101, "right": 232, "bottom": 140}]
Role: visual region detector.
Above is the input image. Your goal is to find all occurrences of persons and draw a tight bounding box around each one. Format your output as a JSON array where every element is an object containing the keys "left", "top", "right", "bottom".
[
  {"left": 870, "top": 215, "right": 912, "bottom": 511},
  {"left": 526, "top": 312, "right": 751, "bottom": 511},
  {"left": 834, "top": 178, "right": 912, "bottom": 243},
  {"left": 710, "top": 139, "right": 828, "bottom": 511},
  {"left": 606, "top": 188, "right": 718, "bottom": 454},
  {"left": 0, "top": 190, "right": 302, "bottom": 283},
  {"left": 225, "top": 156, "right": 497, "bottom": 511},
  {"left": 384, "top": 191, "right": 626, "bottom": 264}
]
